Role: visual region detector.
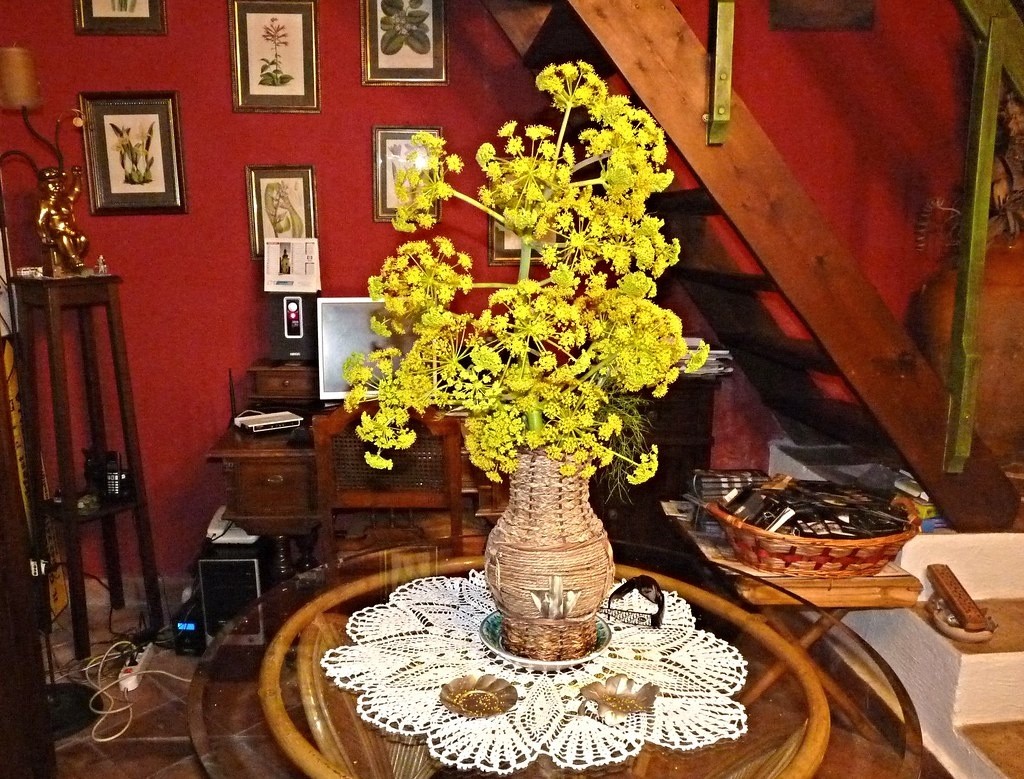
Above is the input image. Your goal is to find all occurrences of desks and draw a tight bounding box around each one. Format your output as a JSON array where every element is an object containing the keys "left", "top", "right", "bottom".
[
  {"left": 730, "top": 554, "right": 920, "bottom": 743},
  {"left": 8, "top": 276, "right": 166, "bottom": 658},
  {"left": 205, "top": 359, "right": 489, "bottom": 565}
]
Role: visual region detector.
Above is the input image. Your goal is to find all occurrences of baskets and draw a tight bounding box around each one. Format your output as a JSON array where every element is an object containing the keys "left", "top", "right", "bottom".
[{"left": 705, "top": 480, "right": 922, "bottom": 579}]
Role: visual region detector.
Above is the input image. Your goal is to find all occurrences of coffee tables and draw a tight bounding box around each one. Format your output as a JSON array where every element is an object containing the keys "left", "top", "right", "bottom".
[{"left": 190, "top": 545, "right": 923, "bottom": 779}]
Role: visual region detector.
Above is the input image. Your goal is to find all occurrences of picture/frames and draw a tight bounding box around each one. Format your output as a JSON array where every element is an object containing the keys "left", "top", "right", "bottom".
[
  {"left": 229, "top": 0, "right": 323, "bottom": 114},
  {"left": 73, "top": 0, "right": 168, "bottom": 37},
  {"left": 244, "top": 164, "right": 321, "bottom": 261},
  {"left": 371, "top": 125, "right": 444, "bottom": 224},
  {"left": 79, "top": 89, "right": 188, "bottom": 214},
  {"left": 360, "top": 0, "right": 449, "bottom": 87},
  {"left": 489, "top": 158, "right": 556, "bottom": 265}
]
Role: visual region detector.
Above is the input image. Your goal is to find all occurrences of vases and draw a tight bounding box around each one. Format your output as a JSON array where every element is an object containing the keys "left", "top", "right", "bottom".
[{"left": 476, "top": 450, "right": 615, "bottom": 665}]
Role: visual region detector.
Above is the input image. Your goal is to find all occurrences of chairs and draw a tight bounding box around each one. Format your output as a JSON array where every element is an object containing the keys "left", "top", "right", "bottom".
[{"left": 314, "top": 397, "right": 464, "bottom": 567}]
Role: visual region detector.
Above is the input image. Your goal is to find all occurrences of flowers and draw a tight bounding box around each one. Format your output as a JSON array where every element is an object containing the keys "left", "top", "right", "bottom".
[{"left": 342, "top": 60, "right": 713, "bottom": 483}]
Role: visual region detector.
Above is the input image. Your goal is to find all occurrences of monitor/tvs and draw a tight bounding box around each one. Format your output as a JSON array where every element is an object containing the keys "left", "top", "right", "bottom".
[{"left": 316, "top": 298, "right": 419, "bottom": 401}]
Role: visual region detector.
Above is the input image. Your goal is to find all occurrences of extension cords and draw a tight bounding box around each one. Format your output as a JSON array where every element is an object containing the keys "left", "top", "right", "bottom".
[
  {"left": 118, "top": 642, "right": 155, "bottom": 692},
  {"left": 927, "top": 564, "right": 988, "bottom": 632}
]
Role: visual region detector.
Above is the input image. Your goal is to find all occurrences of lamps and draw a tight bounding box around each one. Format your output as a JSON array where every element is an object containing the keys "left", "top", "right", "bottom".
[{"left": 0, "top": 41, "right": 102, "bottom": 276}]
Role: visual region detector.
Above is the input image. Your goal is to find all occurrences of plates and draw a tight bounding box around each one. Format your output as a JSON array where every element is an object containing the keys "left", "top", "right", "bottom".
[
  {"left": 580, "top": 674, "right": 660, "bottom": 716},
  {"left": 481, "top": 609, "right": 611, "bottom": 669},
  {"left": 440, "top": 674, "right": 517, "bottom": 718}
]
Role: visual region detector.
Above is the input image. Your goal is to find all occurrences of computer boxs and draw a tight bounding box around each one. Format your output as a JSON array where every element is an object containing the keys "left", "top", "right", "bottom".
[{"left": 197, "top": 541, "right": 283, "bottom": 683}]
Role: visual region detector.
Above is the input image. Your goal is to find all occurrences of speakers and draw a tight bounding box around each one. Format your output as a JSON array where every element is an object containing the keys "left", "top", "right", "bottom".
[
  {"left": 173, "top": 601, "right": 207, "bottom": 657},
  {"left": 269, "top": 291, "right": 319, "bottom": 362}
]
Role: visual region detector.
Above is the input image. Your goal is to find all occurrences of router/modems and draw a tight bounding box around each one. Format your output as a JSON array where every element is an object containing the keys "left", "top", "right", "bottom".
[{"left": 229, "top": 367, "right": 304, "bottom": 433}]
